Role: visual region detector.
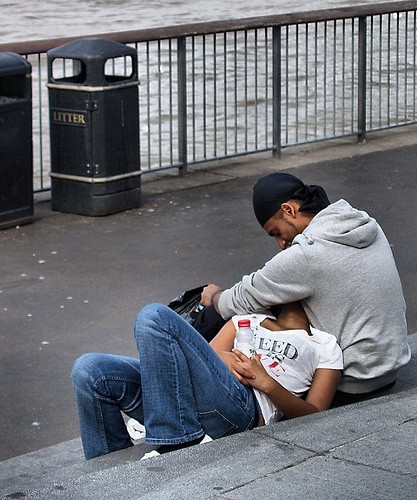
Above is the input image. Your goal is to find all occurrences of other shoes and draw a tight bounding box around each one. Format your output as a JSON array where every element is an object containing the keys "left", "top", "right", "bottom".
[{"left": 141, "top": 435, "right": 213, "bottom": 461}]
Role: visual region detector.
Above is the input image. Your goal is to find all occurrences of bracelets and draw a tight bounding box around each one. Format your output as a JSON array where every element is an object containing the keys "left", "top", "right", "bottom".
[{"left": 210, "top": 289, "right": 224, "bottom": 305}]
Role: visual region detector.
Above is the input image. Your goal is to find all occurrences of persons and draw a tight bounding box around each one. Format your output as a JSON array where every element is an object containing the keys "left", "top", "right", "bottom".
[
  {"left": 192, "top": 172, "right": 412, "bottom": 411},
  {"left": 70, "top": 301, "right": 344, "bottom": 463}
]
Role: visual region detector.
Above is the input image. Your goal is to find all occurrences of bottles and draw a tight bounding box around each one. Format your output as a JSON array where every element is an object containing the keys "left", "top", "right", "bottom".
[{"left": 236, "top": 319, "right": 255, "bottom": 358}]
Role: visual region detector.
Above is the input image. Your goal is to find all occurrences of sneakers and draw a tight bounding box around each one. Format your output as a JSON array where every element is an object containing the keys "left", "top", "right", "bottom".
[{"left": 127, "top": 418, "right": 146, "bottom": 440}]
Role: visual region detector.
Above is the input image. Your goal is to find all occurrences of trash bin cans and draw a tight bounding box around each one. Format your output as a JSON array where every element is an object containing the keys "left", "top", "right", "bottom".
[
  {"left": 0, "top": 51, "right": 34, "bottom": 231},
  {"left": 45, "top": 36, "right": 142, "bottom": 219}
]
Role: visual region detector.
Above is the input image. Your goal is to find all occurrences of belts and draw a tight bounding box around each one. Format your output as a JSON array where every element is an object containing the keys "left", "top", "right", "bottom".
[{"left": 251, "top": 390, "right": 259, "bottom": 429}]
